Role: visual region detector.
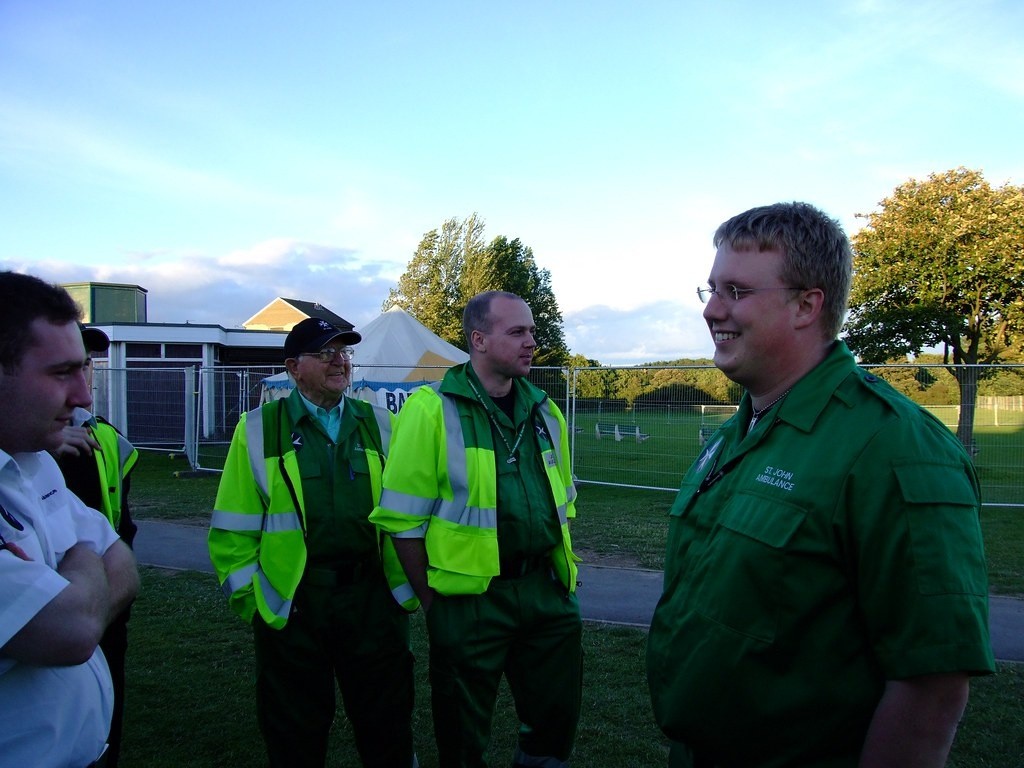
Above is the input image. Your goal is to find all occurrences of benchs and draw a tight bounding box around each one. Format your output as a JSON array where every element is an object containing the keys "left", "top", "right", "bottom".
[
  {"left": 698, "top": 428, "right": 718, "bottom": 446},
  {"left": 595, "top": 422, "right": 650, "bottom": 445}
]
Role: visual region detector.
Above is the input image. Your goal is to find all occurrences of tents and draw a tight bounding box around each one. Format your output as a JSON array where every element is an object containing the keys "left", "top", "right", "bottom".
[{"left": 253, "top": 302, "right": 470, "bottom": 465}]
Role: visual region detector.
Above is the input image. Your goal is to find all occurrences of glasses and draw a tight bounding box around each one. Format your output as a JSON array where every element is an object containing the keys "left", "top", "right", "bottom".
[
  {"left": 299, "top": 347, "right": 354, "bottom": 361},
  {"left": 697, "top": 284, "right": 806, "bottom": 303}
]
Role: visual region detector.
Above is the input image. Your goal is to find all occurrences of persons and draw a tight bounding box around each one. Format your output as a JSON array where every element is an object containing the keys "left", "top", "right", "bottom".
[
  {"left": 55, "top": 320, "right": 141, "bottom": 768},
  {"left": 0, "top": 268, "right": 141, "bottom": 768},
  {"left": 644, "top": 201, "right": 995, "bottom": 768},
  {"left": 206, "top": 316, "right": 418, "bottom": 768},
  {"left": 368, "top": 290, "right": 585, "bottom": 768}
]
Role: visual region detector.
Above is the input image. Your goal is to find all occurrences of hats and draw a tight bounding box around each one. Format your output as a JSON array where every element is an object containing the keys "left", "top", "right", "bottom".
[
  {"left": 283, "top": 318, "right": 362, "bottom": 360},
  {"left": 76, "top": 320, "right": 110, "bottom": 352}
]
